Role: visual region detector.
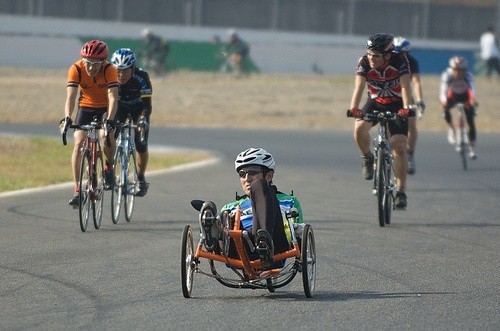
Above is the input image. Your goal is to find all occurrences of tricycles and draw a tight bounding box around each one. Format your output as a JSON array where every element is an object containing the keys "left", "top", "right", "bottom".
[{"left": 180, "top": 199, "right": 318, "bottom": 299}]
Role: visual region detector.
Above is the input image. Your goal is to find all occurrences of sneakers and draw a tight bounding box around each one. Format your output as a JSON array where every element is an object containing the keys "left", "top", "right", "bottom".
[
  {"left": 407, "top": 159, "right": 415, "bottom": 174},
  {"left": 103, "top": 166, "right": 114, "bottom": 189},
  {"left": 68, "top": 192, "right": 84, "bottom": 205},
  {"left": 362, "top": 154, "right": 374, "bottom": 179},
  {"left": 396, "top": 191, "right": 407, "bottom": 208},
  {"left": 135, "top": 177, "right": 146, "bottom": 196}
]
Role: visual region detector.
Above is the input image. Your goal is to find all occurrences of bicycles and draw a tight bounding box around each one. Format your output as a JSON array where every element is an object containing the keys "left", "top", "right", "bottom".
[
  {"left": 442, "top": 102, "right": 472, "bottom": 168},
  {"left": 102, "top": 114, "right": 143, "bottom": 225},
  {"left": 60, "top": 115, "right": 124, "bottom": 231},
  {"left": 347, "top": 109, "right": 417, "bottom": 228}
]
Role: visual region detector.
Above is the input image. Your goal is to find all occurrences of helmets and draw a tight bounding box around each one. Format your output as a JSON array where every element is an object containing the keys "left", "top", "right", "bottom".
[
  {"left": 392, "top": 37, "right": 412, "bottom": 53},
  {"left": 235, "top": 148, "right": 275, "bottom": 172},
  {"left": 366, "top": 34, "right": 395, "bottom": 55},
  {"left": 111, "top": 47, "right": 136, "bottom": 69},
  {"left": 80, "top": 40, "right": 108, "bottom": 58},
  {"left": 449, "top": 56, "right": 467, "bottom": 70}
]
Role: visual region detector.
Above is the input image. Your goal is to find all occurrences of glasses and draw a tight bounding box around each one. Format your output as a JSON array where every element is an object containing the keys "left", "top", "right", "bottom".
[
  {"left": 239, "top": 169, "right": 265, "bottom": 177},
  {"left": 368, "top": 50, "right": 387, "bottom": 57},
  {"left": 82, "top": 58, "right": 104, "bottom": 66}
]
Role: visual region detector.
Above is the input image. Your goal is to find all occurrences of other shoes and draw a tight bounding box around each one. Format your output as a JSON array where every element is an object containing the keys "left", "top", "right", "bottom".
[
  {"left": 199, "top": 201, "right": 219, "bottom": 251},
  {"left": 468, "top": 146, "right": 477, "bottom": 159},
  {"left": 257, "top": 229, "right": 274, "bottom": 268},
  {"left": 448, "top": 129, "right": 457, "bottom": 143}
]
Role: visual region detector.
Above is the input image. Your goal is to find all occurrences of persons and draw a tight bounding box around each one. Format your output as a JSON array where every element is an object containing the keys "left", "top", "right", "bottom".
[
  {"left": 99, "top": 48, "right": 153, "bottom": 196},
  {"left": 351, "top": 33, "right": 418, "bottom": 207},
  {"left": 142, "top": 29, "right": 169, "bottom": 77},
  {"left": 440, "top": 57, "right": 478, "bottom": 158},
  {"left": 222, "top": 32, "right": 249, "bottom": 78},
  {"left": 479, "top": 26, "right": 500, "bottom": 76},
  {"left": 394, "top": 37, "right": 425, "bottom": 173},
  {"left": 60, "top": 40, "right": 120, "bottom": 206},
  {"left": 199, "top": 148, "right": 303, "bottom": 268}
]
